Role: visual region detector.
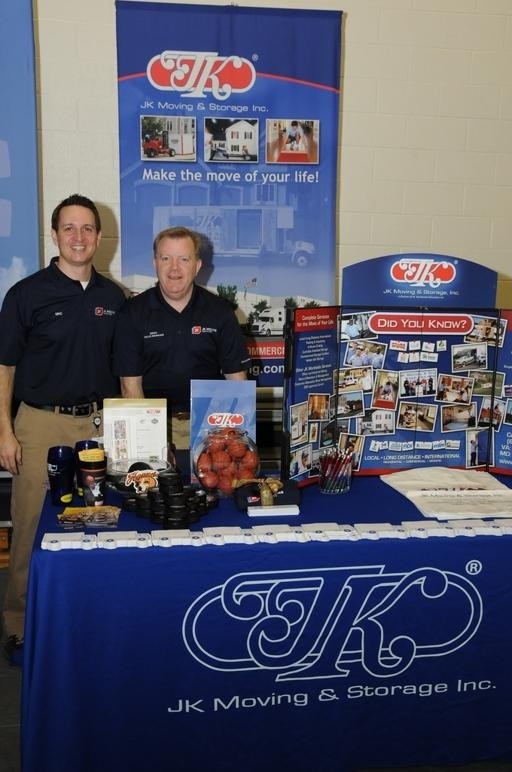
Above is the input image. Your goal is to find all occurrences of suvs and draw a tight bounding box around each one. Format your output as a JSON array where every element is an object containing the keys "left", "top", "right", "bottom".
[{"left": 143, "top": 139, "right": 177, "bottom": 158}]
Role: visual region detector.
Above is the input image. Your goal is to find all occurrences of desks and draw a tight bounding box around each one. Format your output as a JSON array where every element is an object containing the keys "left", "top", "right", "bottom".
[{"left": 21, "top": 472, "right": 512, "bottom": 770}]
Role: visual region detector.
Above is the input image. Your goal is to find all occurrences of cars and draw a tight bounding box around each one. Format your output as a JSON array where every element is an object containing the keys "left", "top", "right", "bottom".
[
  {"left": 479, "top": 382, "right": 497, "bottom": 389},
  {"left": 339, "top": 375, "right": 358, "bottom": 388},
  {"left": 454, "top": 352, "right": 487, "bottom": 366}
]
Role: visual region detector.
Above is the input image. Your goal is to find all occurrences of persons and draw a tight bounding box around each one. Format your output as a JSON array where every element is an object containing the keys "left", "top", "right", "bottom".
[
  {"left": 295, "top": 312, "right": 504, "bottom": 477},
  {"left": 282, "top": 120, "right": 307, "bottom": 152},
  {"left": 108, "top": 224, "right": 253, "bottom": 449},
  {"left": 0, "top": 193, "right": 125, "bottom": 671}
]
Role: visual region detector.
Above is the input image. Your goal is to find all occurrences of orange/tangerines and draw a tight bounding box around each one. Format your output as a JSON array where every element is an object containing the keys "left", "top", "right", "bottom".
[{"left": 198, "top": 437, "right": 258, "bottom": 495}]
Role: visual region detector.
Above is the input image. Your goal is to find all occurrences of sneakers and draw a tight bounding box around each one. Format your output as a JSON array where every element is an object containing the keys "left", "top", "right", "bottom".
[{"left": 2, "top": 634, "right": 25, "bottom": 661}]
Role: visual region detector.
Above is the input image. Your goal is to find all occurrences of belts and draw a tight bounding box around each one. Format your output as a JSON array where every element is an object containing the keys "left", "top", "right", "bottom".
[
  {"left": 168, "top": 410, "right": 191, "bottom": 421},
  {"left": 23, "top": 398, "right": 107, "bottom": 418}
]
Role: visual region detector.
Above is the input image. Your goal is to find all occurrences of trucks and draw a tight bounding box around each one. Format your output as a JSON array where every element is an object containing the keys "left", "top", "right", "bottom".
[{"left": 149, "top": 200, "right": 320, "bottom": 269}]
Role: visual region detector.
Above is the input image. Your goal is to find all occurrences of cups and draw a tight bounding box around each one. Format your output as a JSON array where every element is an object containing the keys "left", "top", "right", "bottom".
[
  {"left": 76, "top": 456, "right": 107, "bottom": 507},
  {"left": 319, "top": 460, "right": 352, "bottom": 495}
]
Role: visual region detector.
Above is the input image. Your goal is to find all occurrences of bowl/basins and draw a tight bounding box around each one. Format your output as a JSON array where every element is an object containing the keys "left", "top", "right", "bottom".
[{"left": 108, "top": 459, "right": 171, "bottom": 495}]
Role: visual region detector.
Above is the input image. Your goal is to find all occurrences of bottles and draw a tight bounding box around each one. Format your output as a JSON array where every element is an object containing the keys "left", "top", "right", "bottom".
[
  {"left": 47, "top": 440, "right": 98, "bottom": 506},
  {"left": 193, "top": 428, "right": 261, "bottom": 499}
]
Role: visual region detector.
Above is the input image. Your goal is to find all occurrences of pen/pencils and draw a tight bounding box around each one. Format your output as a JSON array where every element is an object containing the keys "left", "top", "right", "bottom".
[{"left": 318, "top": 447, "right": 353, "bottom": 494}]
